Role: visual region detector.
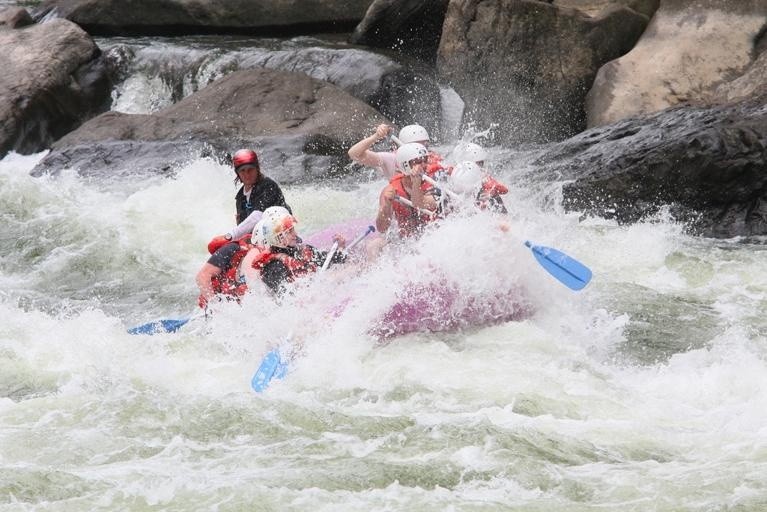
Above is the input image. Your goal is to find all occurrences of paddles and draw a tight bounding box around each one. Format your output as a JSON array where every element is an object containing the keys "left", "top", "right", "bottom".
[
  {"left": 127, "top": 311, "right": 213, "bottom": 334},
  {"left": 252, "top": 241, "right": 339, "bottom": 393},
  {"left": 419, "top": 173, "right": 591, "bottom": 291}
]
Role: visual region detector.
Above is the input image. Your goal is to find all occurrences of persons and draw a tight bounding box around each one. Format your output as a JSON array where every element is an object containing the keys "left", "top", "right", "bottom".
[
  {"left": 196, "top": 207, "right": 347, "bottom": 311},
  {"left": 348, "top": 123, "right": 508, "bottom": 242},
  {"left": 234, "top": 148, "right": 292, "bottom": 226}
]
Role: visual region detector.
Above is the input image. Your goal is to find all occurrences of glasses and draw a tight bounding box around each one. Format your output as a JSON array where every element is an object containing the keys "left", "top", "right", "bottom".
[{"left": 415, "top": 156, "right": 428, "bottom": 164}]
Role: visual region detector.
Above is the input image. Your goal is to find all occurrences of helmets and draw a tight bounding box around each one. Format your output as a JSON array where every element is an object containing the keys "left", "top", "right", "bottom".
[
  {"left": 450, "top": 161, "right": 483, "bottom": 197},
  {"left": 395, "top": 142, "right": 429, "bottom": 177},
  {"left": 233, "top": 148, "right": 259, "bottom": 174},
  {"left": 250, "top": 205, "right": 296, "bottom": 249},
  {"left": 453, "top": 141, "right": 485, "bottom": 168},
  {"left": 398, "top": 124, "right": 429, "bottom": 145}
]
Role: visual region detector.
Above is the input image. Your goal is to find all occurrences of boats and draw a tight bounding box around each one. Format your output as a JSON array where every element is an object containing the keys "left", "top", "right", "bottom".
[{"left": 199, "top": 216, "right": 541, "bottom": 341}]
